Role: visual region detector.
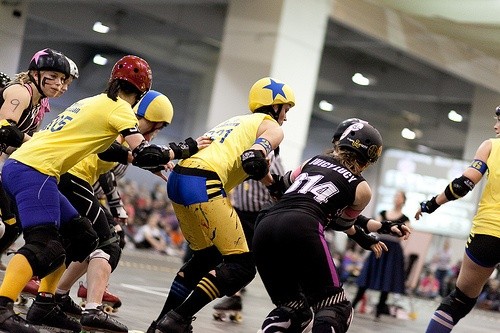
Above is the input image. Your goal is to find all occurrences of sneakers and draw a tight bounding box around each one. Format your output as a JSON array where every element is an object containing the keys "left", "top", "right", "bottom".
[
  {"left": 25, "top": 292, "right": 81, "bottom": 333},
  {"left": 0, "top": 302, "right": 40, "bottom": 333},
  {"left": 80, "top": 305, "right": 128, "bottom": 333},
  {"left": 155, "top": 309, "right": 196, "bottom": 333},
  {"left": 54, "top": 290, "right": 84, "bottom": 320}
]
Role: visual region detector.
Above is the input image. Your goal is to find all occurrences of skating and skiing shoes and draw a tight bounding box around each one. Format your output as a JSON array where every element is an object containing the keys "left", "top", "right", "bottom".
[
  {"left": 13, "top": 275, "right": 41, "bottom": 308},
  {"left": 78, "top": 281, "right": 121, "bottom": 315},
  {"left": 212, "top": 295, "right": 241, "bottom": 323}
]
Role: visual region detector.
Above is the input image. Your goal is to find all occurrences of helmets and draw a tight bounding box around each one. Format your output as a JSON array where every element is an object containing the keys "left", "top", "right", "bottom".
[
  {"left": 338, "top": 122, "right": 382, "bottom": 163},
  {"left": 64, "top": 56, "right": 79, "bottom": 79},
  {"left": 132, "top": 90, "right": 174, "bottom": 124},
  {"left": 333, "top": 118, "right": 369, "bottom": 137},
  {"left": 248, "top": 77, "right": 295, "bottom": 113},
  {"left": 108, "top": 55, "right": 152, "bottom": 92},
  {"left": 27, "top": 48, "right": 70, "bottom": 80},
  {"left": 0, "top": 72, "right": 10, "bottom": 88}
]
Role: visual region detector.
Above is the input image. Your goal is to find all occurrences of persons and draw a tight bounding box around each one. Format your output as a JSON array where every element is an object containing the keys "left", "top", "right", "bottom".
[
  {"left": 0, "top": 48, "right": 500, "bottom": 333},
  {"left": 145, "top": 77, "right": 296, "bottom": 333}
]
